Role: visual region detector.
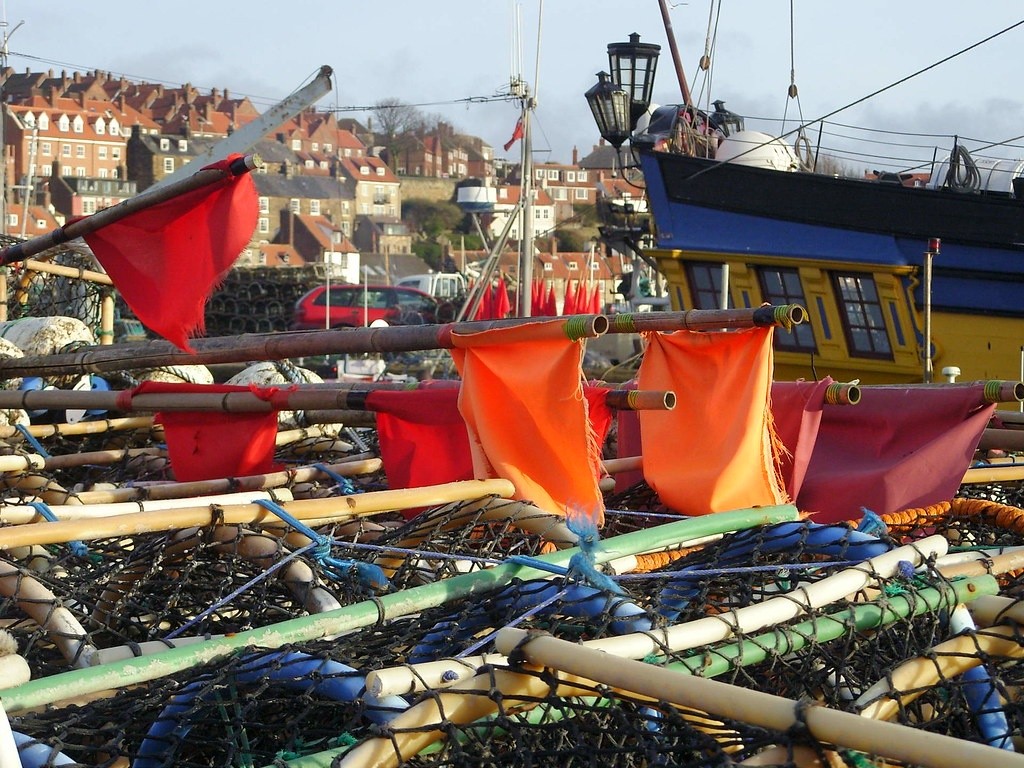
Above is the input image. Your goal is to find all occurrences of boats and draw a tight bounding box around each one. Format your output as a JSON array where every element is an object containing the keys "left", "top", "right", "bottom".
[{"left": 582, "top": 1, "right": 1024, "bottom": 451}]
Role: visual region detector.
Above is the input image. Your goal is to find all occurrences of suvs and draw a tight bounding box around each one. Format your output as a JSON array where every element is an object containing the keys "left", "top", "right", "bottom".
[{"left": 294, "top": 279, "right": 436, "bottom": 330}]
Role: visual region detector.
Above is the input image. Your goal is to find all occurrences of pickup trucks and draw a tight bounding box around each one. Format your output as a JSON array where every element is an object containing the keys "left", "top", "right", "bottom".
[{"left": 395, "top": 270, "right": 468, "bottom": 299}]
